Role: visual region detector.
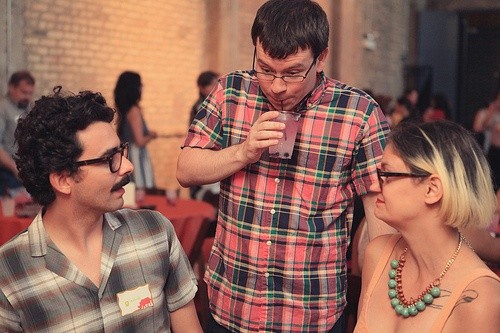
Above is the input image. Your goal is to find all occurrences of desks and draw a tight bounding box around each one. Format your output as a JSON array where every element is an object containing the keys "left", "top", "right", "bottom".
[{"left": 0, "top": 194, "right": 215, "bottom": 282}]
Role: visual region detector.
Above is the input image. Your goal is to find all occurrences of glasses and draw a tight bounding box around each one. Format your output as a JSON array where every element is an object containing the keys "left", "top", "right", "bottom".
[
  {"left": 252, "top": 48, "right": 321, "bottom": 83},
  {"left": 376, "top": 166, "right": 431, "bottom": 185},
  {"left": 70, "top": 142, "right": 130, "bottom": 173}
]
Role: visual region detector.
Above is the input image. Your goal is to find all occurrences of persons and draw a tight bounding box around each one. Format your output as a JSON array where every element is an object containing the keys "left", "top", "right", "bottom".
[
  {"left": 0, "top": 85, "right": 203, "bottom": 333},
  {"left": 176, "top": 0, "right": 399, "bottom": 333},
  {"left": 352, "top": 119, "right": 500, "bottom": 333},
  {"left": 0, "top": 71, "right": 500, "bottom": 203}
]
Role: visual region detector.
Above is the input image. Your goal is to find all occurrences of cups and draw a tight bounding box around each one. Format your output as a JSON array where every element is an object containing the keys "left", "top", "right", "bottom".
[
  {"left": 268, "top": 111, "right": 301, "bottom": 158},
  {"left": 166, "top": 189, "right": 177, "bottom": 205},
  {"left": 2, "top": 198, "right": 14, "bottom": 217}
]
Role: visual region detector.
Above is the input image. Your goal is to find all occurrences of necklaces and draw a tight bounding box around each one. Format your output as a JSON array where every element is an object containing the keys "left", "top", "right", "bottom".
[{"left": 388, "top": 228, "right": 463, "bottom": 317}]
